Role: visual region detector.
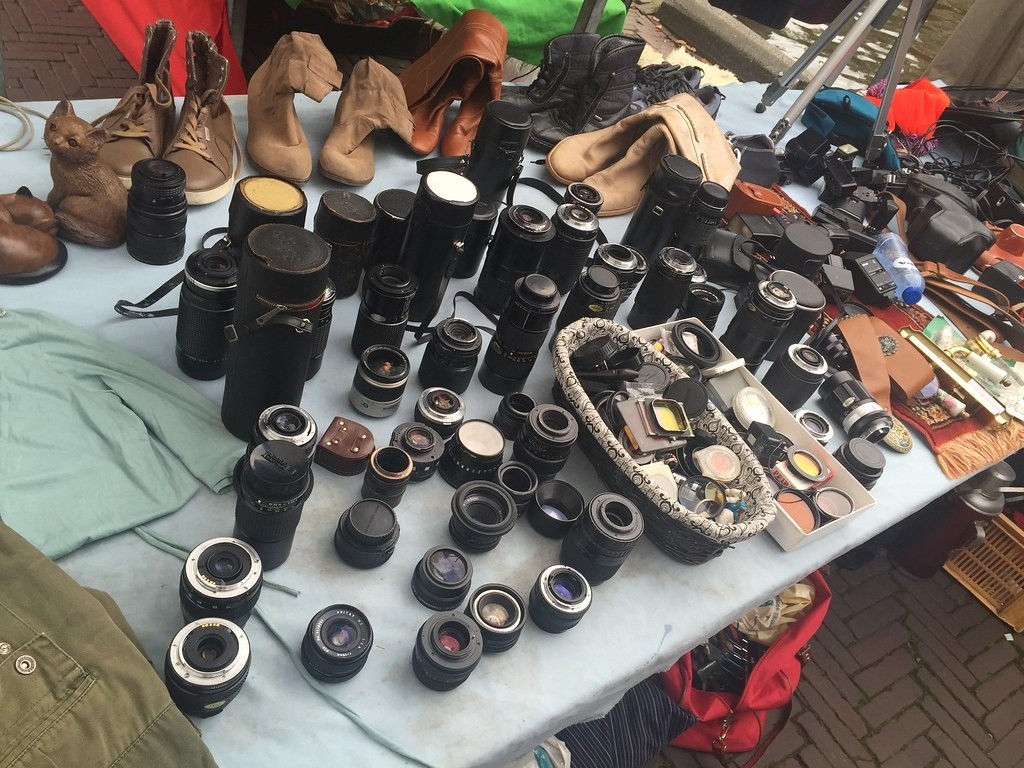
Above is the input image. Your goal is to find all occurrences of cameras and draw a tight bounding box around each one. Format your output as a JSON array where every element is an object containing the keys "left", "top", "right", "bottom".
[{"left": 811, "top": 195, "right": 878, "bottom": 254}]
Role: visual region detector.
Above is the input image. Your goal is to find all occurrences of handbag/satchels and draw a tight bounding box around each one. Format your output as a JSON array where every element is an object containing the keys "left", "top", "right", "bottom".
[
  {"left": 864, "top": 78, "right": 950, "bottom": 155},
  {"left": 703, "top": 229, "right": 757, "bottom": 287},
  {"left": 800, "top": 89, "right": 897, "bottom": 171}
]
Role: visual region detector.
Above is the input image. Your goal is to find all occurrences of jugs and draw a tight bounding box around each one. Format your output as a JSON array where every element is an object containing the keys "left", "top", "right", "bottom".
[{"left": 885, "top": 461, "right": 1024, "bottom": 581}]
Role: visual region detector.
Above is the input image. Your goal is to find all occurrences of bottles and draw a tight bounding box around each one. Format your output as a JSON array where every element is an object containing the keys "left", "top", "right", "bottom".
[{"left": 873, "top": 233, "right": 926, "bottom": 305}]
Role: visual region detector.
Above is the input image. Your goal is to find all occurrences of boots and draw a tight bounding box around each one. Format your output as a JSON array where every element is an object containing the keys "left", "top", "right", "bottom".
[
  {"left": 509, "top": 33, "right": 741, "bottom": 217},
  {"left": 320, "top": 57, "right": 414, "bottom": 185},
  {"left": 398, "top": 10, "right": 506, "bottom": 160},
  {"left": 248, "top": 31, "right": 344, "bottom": 181}
]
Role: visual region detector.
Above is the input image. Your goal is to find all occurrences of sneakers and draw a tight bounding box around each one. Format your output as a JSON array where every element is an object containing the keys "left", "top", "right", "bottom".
[{"left": 89, "top": 19, "right": 242, "bottom": 206}]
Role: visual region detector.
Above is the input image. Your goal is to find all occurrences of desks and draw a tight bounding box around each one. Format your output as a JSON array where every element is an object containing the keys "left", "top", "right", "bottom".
[{"left": 2, "top": 81, "right": 1024, "bottom": 768}]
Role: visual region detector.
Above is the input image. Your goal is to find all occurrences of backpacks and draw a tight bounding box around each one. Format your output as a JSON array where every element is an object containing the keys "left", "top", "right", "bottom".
[{"left": 663, "top": 567, "right": 834, "bottom": 763}]
